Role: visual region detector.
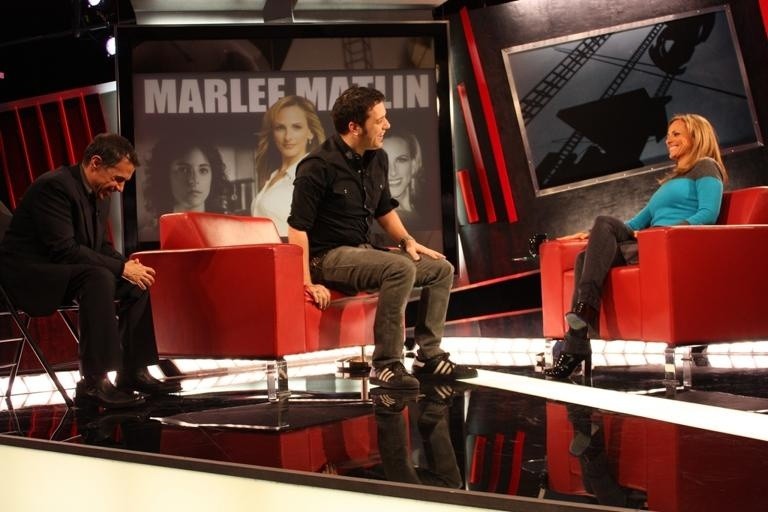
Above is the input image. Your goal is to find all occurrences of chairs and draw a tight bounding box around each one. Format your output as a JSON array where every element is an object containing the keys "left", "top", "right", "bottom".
[{"left": 0, "top": 198, "right": 122, "bottom": 410}]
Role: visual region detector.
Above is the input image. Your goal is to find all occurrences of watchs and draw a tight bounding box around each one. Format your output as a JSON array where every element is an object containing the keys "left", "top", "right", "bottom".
[{"left": 398, "top": 236, "right": 415, "bottom": 249}]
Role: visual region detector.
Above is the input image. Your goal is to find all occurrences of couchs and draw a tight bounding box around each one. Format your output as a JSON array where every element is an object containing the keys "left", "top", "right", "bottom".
[
  {"left": 125, "top": 211, "right": 400, "bottom": 433},
  {"left": 546, "top": 390, "right": 768, "bottom": 511},
  {"left": 537, "top": 185, "right": 768, "bottom": 398},
  {"left": 159, "top": 396, "right": 383, "bottom": 475}
]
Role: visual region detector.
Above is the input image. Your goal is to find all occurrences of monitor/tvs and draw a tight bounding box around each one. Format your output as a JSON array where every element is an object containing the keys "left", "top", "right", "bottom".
[{"left": 501, "top": 4, "right": 763, "bottom": 198}]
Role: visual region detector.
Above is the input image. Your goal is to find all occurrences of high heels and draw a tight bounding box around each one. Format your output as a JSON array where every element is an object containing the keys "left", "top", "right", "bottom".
[
  {"left": 563, "top": 303, "right": 600, "bottom": 340},
  {"left": 542, "top": 353, "right": 592, "bottom": 377}
]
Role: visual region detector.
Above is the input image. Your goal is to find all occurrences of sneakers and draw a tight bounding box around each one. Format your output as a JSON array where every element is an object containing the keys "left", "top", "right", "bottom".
[
  {"left": 369, "top": 385, "right": 419, "bottom": 409},
  {"left": 368, "top": 361, "right": 420, "bottom": 390},
  {"left": 421, "top": 381, "right": 476, "bottom": 399},
  {"left": 412, "top": 353, "right": 477, "bottom": 380}
]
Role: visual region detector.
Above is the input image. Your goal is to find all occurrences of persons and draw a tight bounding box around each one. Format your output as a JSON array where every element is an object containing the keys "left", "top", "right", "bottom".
[
  {"left": 79, "top": 392, "right": 176, "bottom": 454},
  {"left": 0, "top": 132, "right": 182, "bottom": 409},
  {"left": 141, "top": 127, "right": 231, "bottom": 242},
  {"left": 545, "top": 113, "right": 730, "bottom": 376},
  {"left": 251, "top": 96, "right": 324, "bottom": 243},
  {"left": 371, "top": 123, "right": 425, "bottom": 233},
  {"left": 319, "top": 381, "right": 476, "bottom": 492},
  {"left": 567, "top": 404, "right": 648, "bottom": 511},
  {"left": 287, "top": 84, "right": 477, "bottom": 391}
]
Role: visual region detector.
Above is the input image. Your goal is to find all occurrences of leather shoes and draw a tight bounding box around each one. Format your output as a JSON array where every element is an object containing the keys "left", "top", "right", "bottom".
[
  {"left": 75, "top": 380, "right": 147, "bottom": 412},
  {"left": 117, "top": 366, "right": 183, "bottom": 397}
]
